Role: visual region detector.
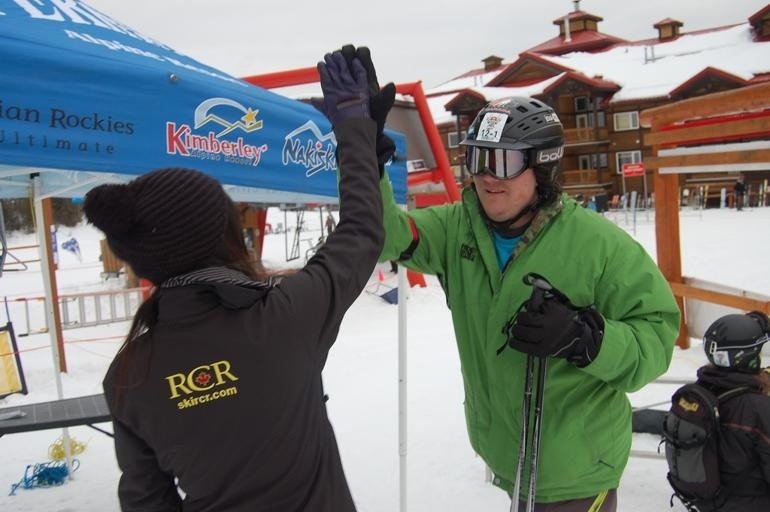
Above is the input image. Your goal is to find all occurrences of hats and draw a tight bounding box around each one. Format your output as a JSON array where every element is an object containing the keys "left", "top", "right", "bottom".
[{"left": 83, "top": 169, "right": 245, "bottom": 285}]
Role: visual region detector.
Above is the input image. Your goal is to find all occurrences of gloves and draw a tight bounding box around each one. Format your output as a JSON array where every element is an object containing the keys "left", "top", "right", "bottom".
[
  {"left": 310, "top": 51, "right": 371, "bottom": 128},
  {"left": 333, "top": 45, "right": 395, "bottom": 164},
  {"left": 509, "top": 295, "right": 603, "bottom": 368}
]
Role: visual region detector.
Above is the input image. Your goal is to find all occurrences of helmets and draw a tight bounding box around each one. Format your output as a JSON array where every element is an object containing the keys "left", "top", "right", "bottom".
[
  {"left": 459, "top": 97, "right": 565, "bottom": 150},
  {"left": 704, "top": 311, "right": 770, "bottom": 375}
]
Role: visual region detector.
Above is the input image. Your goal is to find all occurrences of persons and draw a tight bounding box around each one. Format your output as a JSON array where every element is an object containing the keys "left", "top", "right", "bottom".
[
  {"left": 663, "top": 311, "right": 770, "bottom": 512},
  {"left": 344, "top": 46, "right": 682, "bottom": 512},
  {"left": 734, "top": 179, "right": 746, "bottom": 211},
  {"left": 83, "top": 51, "right": 385, "bottom": 512}
]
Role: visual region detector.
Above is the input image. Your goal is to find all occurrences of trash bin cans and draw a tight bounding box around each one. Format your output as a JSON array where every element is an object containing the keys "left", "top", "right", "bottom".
[{"left": 596, "top": 194, "right": 608, "bottom": 212}]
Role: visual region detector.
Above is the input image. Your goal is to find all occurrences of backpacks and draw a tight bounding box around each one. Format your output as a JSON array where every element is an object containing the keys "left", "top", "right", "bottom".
[{"left": 662, "top": 378, "right": 760, "bottom": 512}]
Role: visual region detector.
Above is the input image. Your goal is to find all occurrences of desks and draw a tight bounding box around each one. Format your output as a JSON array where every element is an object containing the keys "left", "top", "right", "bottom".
[{"left": 1, "top": 393, "right": 115, "bottom": 439}]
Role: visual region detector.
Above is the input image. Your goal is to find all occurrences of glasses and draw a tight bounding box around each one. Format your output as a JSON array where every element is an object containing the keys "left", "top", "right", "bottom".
[{"left": 466, "top": 145, "right": 564, "bottom": 180}]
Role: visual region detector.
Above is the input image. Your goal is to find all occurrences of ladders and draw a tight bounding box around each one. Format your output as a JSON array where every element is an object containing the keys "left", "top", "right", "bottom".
[{"left": 14, "top": 287, "right": 153, "bottom": 337}]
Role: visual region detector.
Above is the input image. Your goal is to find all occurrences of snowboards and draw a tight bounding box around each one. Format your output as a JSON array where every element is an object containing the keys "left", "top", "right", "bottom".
[
  {"left": 720, "top": 187, "right": 727, "bottom": 209},
  {"left": 612, "top": 191, "right": 653, "bottom": 214},
  {"left": 681, "top": 188, "right": 690, "bottom": 211}
]
